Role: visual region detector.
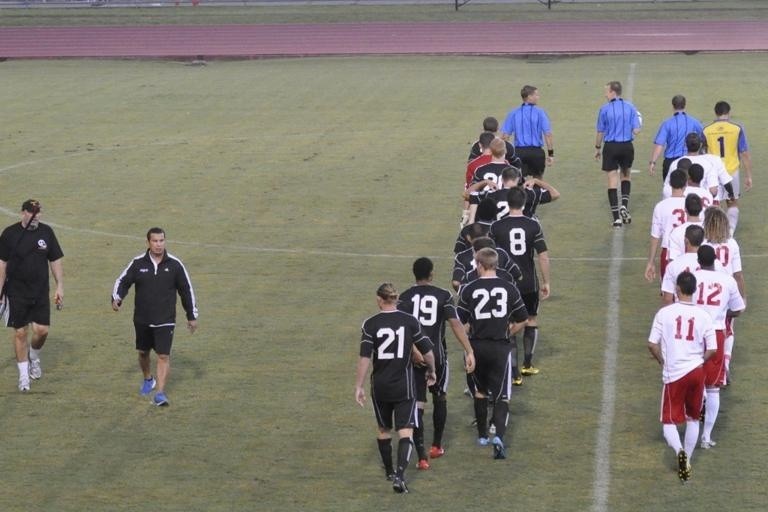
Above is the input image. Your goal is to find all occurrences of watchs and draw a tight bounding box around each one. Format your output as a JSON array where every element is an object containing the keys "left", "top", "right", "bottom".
[
  {"left": 595, "top": 145, "right": 601, "bottom": 149},
  {"left": 650, "top": 160, "right": 656, "bottom": 164}
]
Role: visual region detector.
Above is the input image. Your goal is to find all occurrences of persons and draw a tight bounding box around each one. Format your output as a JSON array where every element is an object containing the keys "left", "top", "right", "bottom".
[
  {"left": 648, "top": 94, "right": 707, "bottom": 185},
  {"left": 500, "top": 86, "right": 562, "bottom": 182},
  {"left": 666, "top": 192, "right": 705, "bottom": 260},
  {"left": 701, "top": 100, "right": 751, "bottom": 239},
  {"left": 393, "top": 256, "right": 476, "bottom": 471},
  {"left": 455, "top": 248, "right": 529, "bottom": 461},
  {"left": 662, "top": 225, "right": 703, "bottom": 307},
  {"left": 593, "top": 82, "right": 642, "bottom": 228},
  {"left": 468, "top": 119, "right": 521, "bottom": 168},
  {"left": 692, "top": 247, "right": 744, "bottom": 451},
  {"left": 459, "top": 239, "right": 520, "bottom": 403},
  {"left": 461, "top": 168, "right": 560, "bottom": 220},
  {"left": 699, "top": 208, "right": 745, "bottom": 388},
  {"left": 663, "top": 132, "right": 735, "bottom": 205},
  {"left": 110, "top": 228, "right": 199, "bottom": 406},
  {"left": 488, "top": 188, "right": 551, "bottom": 388},
  {"left": 454, "top": 198, "right": 501, "bottom": 254},
  {"left": 353, "top": 283, "right": 439, "bottom": 492},
  {"left": 685, "top": 165, "right": 714, "bottom": 205},
  {"left": 647, "top": 270, "right": 718, "bottom": 481},
  {"left": 676, "top": 158, "right": 693, "bottom": 174},
  {"left": 1, "top": 199, "right": 63, "bottom": 392},
  {"left": 468, "top": 138, "right": 520, "bottom": 221},
  {"left": 451, "top": 224, "right": 523, "bottom": 293},
  {"left": 459, "top": 132, "right": 512, "bottom": 224},
  {"left": 644, "top": 169, "right": 688, "bottom": 285}
]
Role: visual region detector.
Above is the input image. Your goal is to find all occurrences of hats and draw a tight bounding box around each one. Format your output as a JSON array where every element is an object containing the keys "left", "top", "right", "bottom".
[{"left": 22, "top": 200, "right": 41, "bottom": 210}]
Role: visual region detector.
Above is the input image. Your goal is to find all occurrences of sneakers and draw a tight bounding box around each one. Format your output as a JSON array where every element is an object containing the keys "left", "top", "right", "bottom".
[
  {"left": 620, "top": 207, "right": 631, "bottom": 225},
  {"left": 140, "top": 378, "right": 156, "bottom": 394},
  {"left": 701, "top": 438, "right": 716, "bottom": 448},
  {"left": 614, "top": 219, "right": 622, "bottom": 228},
  {"left": 521, "top": 366, "right": 539, "bottom": 375},
  {"left": 480, "top": 438, "right": 488, "bottom": 446},
  {"left": 677, "top": 450, "right": 689, "bottom": 483},
  {"left": 493, "top": 437, "right": 506, "bottom": 459},
  {"left": 429, "top": 447, "right": 443, "bottom": 458},
  {"left": 513, "top": 378, "right": 522, "bottom": 385},
  {"left": 28, "top": 353, "right": 41, "bottom": 380},
  {"left": 153, "top": 393, "right": 169, "bottom": 406},
  {"left": 19, "top": 375, "right": 30, "bottom": 391},
  {"left": 387, "top": 471, "right": 396, "bottom": 481},
  {"left": 392, "top": 477, "right": 408, "bottom": 494},
  {"left": 416, "top": 460, "right": 430, "bottom": 471}
]
